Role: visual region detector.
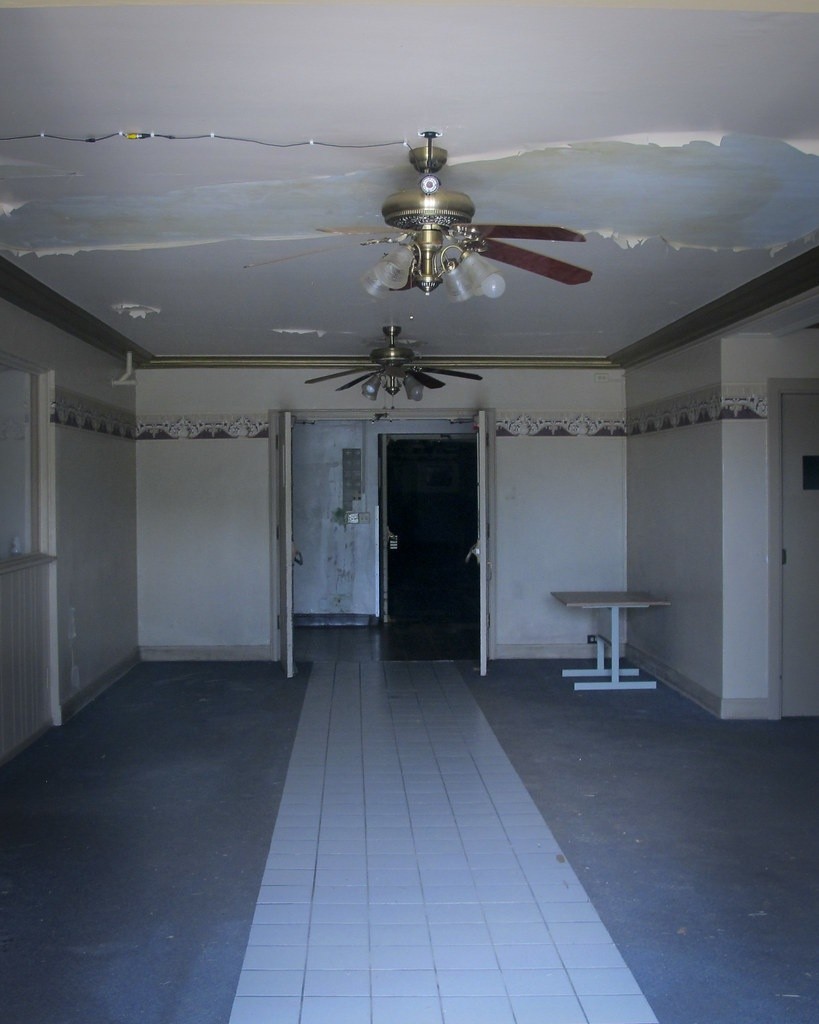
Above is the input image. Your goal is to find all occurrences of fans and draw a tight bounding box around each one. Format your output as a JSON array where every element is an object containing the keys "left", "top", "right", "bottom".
[
  {"left": 244, "top": 131, "right": 593, "bottom": 285},
  {"left": 304, "top": 325, "right": 483, "bottom": 391}
]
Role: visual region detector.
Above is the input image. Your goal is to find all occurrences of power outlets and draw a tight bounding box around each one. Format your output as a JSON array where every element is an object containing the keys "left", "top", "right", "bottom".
[{"left": 347, "top": 513, "right": 358, "bottom": 523}]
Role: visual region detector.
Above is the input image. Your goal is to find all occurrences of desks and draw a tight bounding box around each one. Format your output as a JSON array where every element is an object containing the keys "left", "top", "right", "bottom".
[{"left": 550, "top": 591, "right": 671, "bottom": 690}]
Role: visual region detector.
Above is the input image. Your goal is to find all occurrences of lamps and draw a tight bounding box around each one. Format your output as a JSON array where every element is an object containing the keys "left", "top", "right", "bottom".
[
  {"left": 360, "top": 234, "right": 505, "bottom": 302},
  {"left": 362, "top": 366, "right": 424, "bottom": 401}
]
